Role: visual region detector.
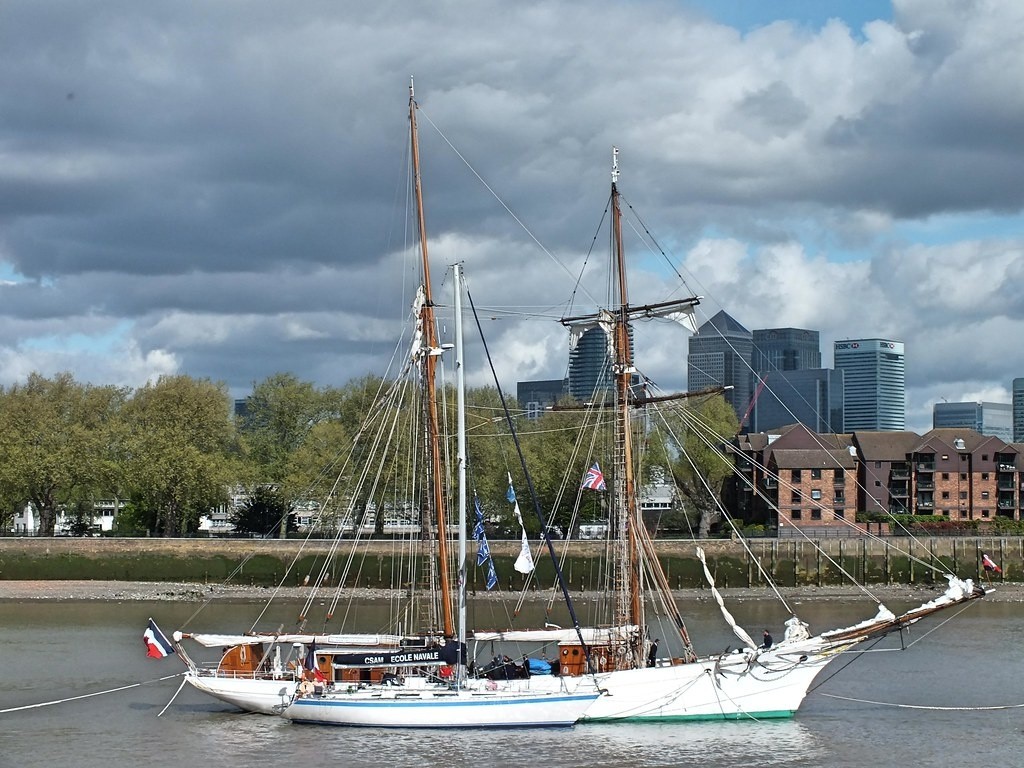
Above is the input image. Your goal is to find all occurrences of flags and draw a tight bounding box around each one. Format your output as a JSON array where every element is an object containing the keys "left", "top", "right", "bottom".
[
  {"left": 304, "top": 637, "right": 329, "bottom": 686},
  {"left": 582, "top": 461, "right": 608, "bottom": 492},
  {"left": 143, "top": 619, "right": 175, "bottom": 659},
  {"left": 471, "top": 495, "right": 497, "bottom": 590},
  {"left": 505, "top": 472, "right": 535, "bottom": 574}
]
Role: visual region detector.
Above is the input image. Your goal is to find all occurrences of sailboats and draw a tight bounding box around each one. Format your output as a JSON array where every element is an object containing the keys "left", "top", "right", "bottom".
[
  {"left": 169, "top": 65, "right": 1001, "bottom": 721},
  {"left": 276, "top": 260, "right": 607, "bottom": 729}
]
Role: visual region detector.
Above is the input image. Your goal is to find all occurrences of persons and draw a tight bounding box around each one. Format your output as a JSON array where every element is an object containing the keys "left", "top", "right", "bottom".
[
  {"left": 468, "top": 653, "right": 531, "bottom": 681},
  {"left": 648, "top": 639, "right": 660, "bottom": 666},
  {"left": 757, "top": 630, "right": 772, "bottom": 650}
]
